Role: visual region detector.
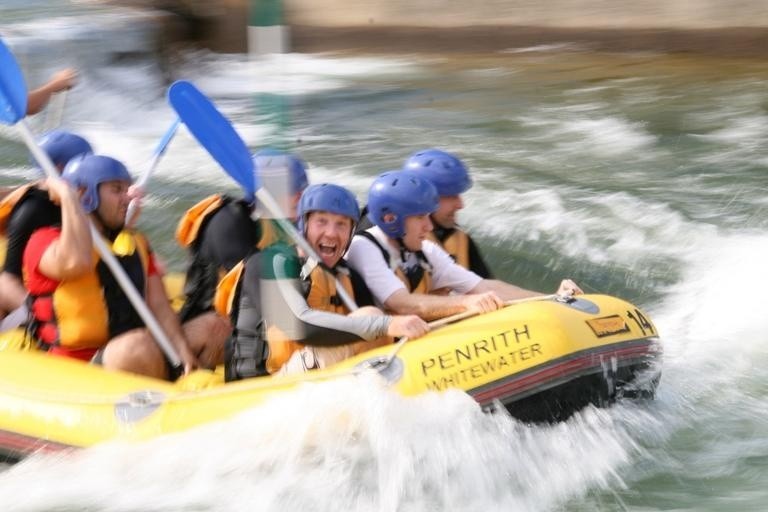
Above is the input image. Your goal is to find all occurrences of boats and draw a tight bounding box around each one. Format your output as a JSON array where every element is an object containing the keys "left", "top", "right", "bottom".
[{"left": 0, "top": 271, "right": 665, "bottom": 462}]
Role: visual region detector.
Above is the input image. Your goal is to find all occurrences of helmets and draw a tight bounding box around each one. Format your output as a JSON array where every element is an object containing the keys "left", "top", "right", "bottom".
[
  {"left": 29, "top": 132, "right": 136, "bottom": 212},
  {"left": 251, "top": 151, "right": 360, "bottom": 255},
  {"left": 366, "top": 150, "right": 474, "bottom": 239}
]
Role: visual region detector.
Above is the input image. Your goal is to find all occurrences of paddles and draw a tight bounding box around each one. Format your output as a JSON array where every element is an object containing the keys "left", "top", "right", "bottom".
[
  {"left": 126, "top": 115, "right": 181, "bottom": 226},
  {"left": 167, "top": 81, "right": 357, "bottom": 310},
  {"left": 0, "top": 39, "right": 180, "bottom": 369}
]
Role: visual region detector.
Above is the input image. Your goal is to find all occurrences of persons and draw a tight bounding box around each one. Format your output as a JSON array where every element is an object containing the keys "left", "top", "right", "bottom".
[
  {"left": 403, "top": 150, "right": 492, "bottom": 279},
  {"left": 176, "top": 149, "right": 308, "bottom": 369},
  {"left": 234, "top": 183, "right": 431, "bottom": 378},
  {"left": 21, "top": 152, "right": 234, "bottom": 380},
  {"left": 27, "top": 69, "right": 76, "bottom": 115},
  {"left": 342, "top": 170, "right": 585, "bottom": 322},
  {"left": 0, "top": 127, "right": 143, "bottom": 327}
]
